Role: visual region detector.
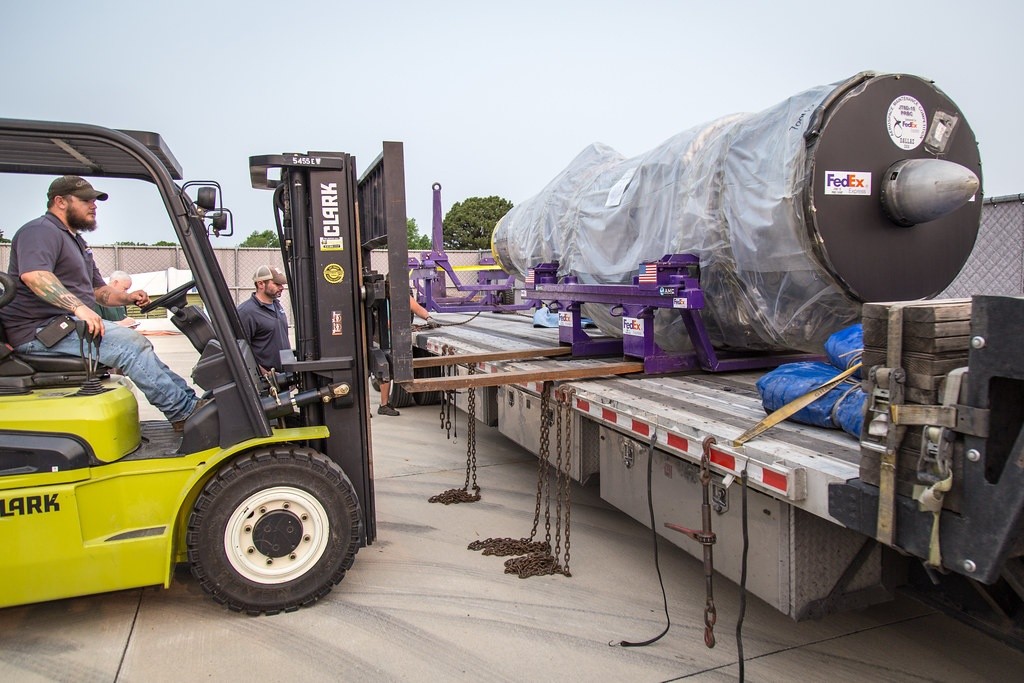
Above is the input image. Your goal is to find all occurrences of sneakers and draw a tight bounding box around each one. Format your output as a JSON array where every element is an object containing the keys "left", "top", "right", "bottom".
[{"left": 378, "top": 404, "right": 399, "bottom": 415}]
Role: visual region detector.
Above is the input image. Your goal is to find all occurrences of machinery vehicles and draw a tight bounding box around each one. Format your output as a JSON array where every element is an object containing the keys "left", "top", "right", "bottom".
[{"left": 0, "top": 118, "right": 643, "bottom": 618}]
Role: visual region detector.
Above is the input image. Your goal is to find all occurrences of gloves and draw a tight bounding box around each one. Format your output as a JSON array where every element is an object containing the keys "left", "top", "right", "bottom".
[{"left": 427, "top": 318, "right": 438, "bottom": 329}]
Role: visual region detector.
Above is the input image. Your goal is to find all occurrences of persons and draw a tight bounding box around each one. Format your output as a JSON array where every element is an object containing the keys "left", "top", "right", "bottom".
[
  {"left": 90, "top": 269, "right": 137, "bottom": 374},
  {"left": 235, "top": 264, "right": 292, "bottom": 393},
  {"left": 367, "top": 272, "right": 438, "bottom": 418},
  {"left": 0, "top": 176, "right": 207, "bottom": 433}
]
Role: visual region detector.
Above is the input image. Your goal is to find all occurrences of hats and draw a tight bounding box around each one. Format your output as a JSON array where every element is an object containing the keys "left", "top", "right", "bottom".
[
  {"left": 47, "top": 176, "right": 108, "bottom": 201},
  {"left": 252, "top": 265, "right": 287, "bottom": 284}
]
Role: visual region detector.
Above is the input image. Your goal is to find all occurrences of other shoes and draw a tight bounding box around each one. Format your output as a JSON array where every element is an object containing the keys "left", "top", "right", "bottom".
[{"left": 172, "top": 399, "right": 207, "bottom": 432}]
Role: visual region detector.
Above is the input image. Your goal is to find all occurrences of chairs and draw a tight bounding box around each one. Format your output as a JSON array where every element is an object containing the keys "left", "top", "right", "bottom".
[{"left": 0, "top": 272, "right": 111, "bottom": 392}]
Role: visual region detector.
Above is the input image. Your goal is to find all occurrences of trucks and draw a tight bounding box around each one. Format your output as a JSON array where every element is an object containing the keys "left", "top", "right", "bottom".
[{"left": 388, "top": 278, "right": 1024, "bottom": 651}]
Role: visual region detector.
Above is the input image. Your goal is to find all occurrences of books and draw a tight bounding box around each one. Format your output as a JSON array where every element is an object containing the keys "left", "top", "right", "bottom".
[{"left": 127, "top": 321, "right": 140, "bottom": 328}]
